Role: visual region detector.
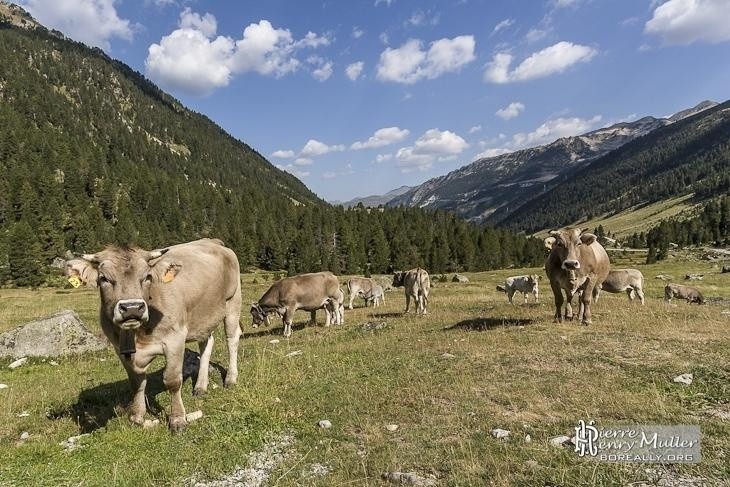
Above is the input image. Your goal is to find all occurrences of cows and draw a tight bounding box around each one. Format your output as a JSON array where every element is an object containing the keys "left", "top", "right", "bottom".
[
  {"left": 391, "top": 267, "right": 431, "bottom": 317},
  {"left": 324, "top": 289, "right": 345, "bottom": 327},
  {"left": 246, "top": 271, "right": 341, "bottom": 337},
  {"left": 665, "top": 283, "right": 706, "bottom": 305},
  {"left": 544, "top": 227, "right": 610, "bottom": 326},
  {"left": 366, "top": 285, "right": 386, "bottom": 308},
  {"left": 347, "top": 278, "right": 377, "bottom": 310},
  {"left": 577, "top": 269, "right": 644, "bottom": 306},
  {"left": 505, "top": 274, "right": 543, "bottom": 306},
  {"left": 67, "top": 237, "right": 245, "bottom": 434}
]
